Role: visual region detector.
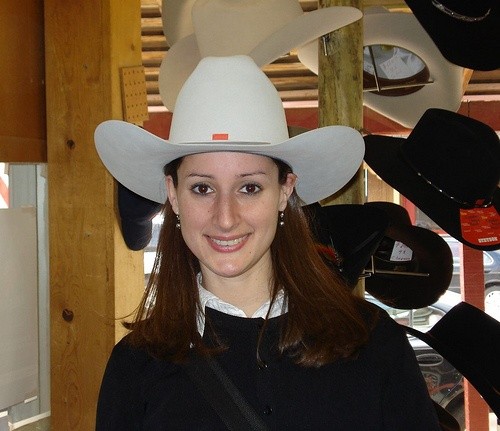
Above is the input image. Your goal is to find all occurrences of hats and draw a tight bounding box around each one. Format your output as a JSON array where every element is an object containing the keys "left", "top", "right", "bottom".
[
  {"left": 361, "top": 108, "right": 500, "bottom": 250},
  {"left": 300, "top": 200, "right": 379, "bottom": 291},
  {"left": 290, "top": 1, "right": 462, "bottom": 128},
  {"left": 396, "top": 300, "right": 500, "bottom": 424},
  {"left": 361, "top": 200, "right": 454, "bottom": 309},
  {"left": 116, "top": 182, "right": 163, "bottom": 251},
  {"left": 95, "top": 55, "right": 364, "bottom": 207},
  {"left": 159, "top": 0, "right": 363, "bottom": 110},
  {"left": 404, "top": 0, "right": 499, "bottom": 69}
]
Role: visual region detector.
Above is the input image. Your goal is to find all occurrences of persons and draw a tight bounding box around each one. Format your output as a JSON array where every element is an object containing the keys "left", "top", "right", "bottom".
[{"left": 95, "top": 55, "right": 461, "bottom": 431}]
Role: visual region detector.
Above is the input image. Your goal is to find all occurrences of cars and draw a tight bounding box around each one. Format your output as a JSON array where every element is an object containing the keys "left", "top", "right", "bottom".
[
  {"left": 364, "top": 289, "right": 465, "bottom": 431},
  {"left": 438, "top": 233, "right": 500, "bottom": 306}
]
